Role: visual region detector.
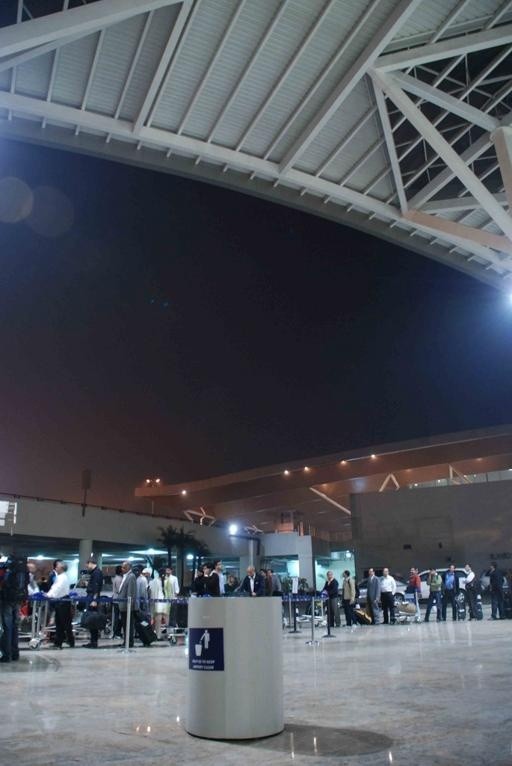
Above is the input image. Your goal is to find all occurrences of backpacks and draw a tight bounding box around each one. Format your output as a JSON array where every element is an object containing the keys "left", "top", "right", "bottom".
[{"left": 348, "top": 579, "right": 359, "bottom": 598}]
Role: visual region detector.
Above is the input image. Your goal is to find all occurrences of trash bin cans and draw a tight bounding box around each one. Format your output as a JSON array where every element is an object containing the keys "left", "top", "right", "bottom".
[{"left": 186, "top": 596, "right": 284, "bottom": 740}]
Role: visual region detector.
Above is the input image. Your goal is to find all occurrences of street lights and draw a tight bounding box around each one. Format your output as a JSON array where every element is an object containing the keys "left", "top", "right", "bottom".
[{"left": 145, "top": 476, "right": 161, "bottom": 513}]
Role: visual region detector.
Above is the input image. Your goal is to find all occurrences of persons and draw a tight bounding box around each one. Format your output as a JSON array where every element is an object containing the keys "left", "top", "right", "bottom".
[
  {"left": 366, "top": 567, "right": 381, "bottom": 625},
  {"left": 406, "top": 566, "right": 422, "bottom": 600},
  {"left": 341, "top": 570, "right": 357, "bottom": 627},
  {"left": 322, "top": 570, "right": 341, "bottom": 628},
  {"left": 297, "top": 576, "right": 311, "bottom": 597},
  {"left": 462, "top": 563, "right": 481, "bottom": 621},
  {"left": 192, "top": 559, "right": 282, "bottom": 598},
  {"left": 424, "top": 567, "right": 443, "bottom": 622},
  {"left": 485, "top": 562, "right": 504, "bottom": 621},
  {"left": 0, "top": 551, "right": 182, "bottom": 664},
  {"left": 441, "top": 563, "right": 460, "bottom": 622},
  {"left": 379, "top": 568, "right": 397, "bottom": 624}
]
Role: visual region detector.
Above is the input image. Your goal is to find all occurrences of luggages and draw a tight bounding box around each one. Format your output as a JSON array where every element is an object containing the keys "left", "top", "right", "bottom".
[
  {"left": 458, "top": 608, "right": 465, "bottom": 619},
  {"left": 135, "top": 618, "right": 157, "bottom": 644},
  {"left": 351, "top": 609, "right": 372, "bottom": 625}
]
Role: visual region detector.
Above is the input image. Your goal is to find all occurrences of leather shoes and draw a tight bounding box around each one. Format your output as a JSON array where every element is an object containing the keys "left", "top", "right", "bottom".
[{"left": 83, "top": 643, "right": 97, "bottom": 647}]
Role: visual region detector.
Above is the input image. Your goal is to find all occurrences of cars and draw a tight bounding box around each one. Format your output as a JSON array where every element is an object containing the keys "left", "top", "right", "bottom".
[{"left": 337, "top": 575, "right": 410, "bottom": 611}]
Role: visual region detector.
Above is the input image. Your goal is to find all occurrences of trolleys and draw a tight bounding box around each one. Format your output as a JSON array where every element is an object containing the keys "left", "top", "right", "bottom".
[
  {"left": 297, "top": 591, "right": 329, "bottom": 629},
  {"left": 27, "top": 618, "right": 90, "bottom": 650},
  {"left": 393, "top": 587, "right": 423, "bottom": 624},
  {"left": 159, "top": 590, "right": 196, "bottom": 646}
]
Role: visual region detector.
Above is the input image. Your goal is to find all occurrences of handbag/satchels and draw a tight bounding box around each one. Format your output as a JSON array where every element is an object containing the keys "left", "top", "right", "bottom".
[{"left": 81, "top": 607, "right": 107, "bottom": 629}]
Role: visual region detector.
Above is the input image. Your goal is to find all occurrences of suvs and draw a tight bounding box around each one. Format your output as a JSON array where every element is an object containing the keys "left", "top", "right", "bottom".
[
  {"left": 403, "top": 567, "right": 471, "bottom": 605},
  {"left": 478, "top": 569, "right": 512, "bottom": 605},
  {"left": 64, "top": 571, "right": 115, "bottom": 612}
]
